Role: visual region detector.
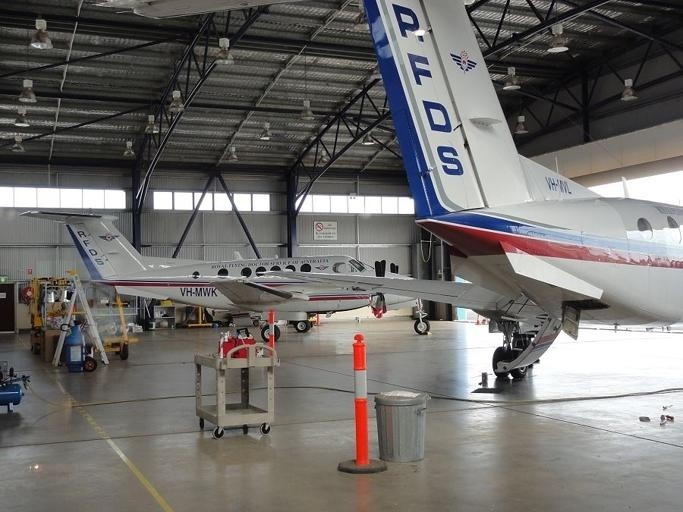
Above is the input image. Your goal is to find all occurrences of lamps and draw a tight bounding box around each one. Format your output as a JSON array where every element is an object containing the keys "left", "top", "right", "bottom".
[
  {"left": 4, "top": 0, "right": 53, "bottom": 153},
  {"left": 362, "top": 133, "right": 374, "bottom": 146},
  {"left": 503, "top": 0, "right": 568, "bottom": 135},
  {"left": 617, "top": 1, "right": 640, "bottom": 103},
  {"left": 318, "top": 148, "right": 330, "bottom": 166},
  {"left": 215, "top": 12, "right": 236, "bottom": 66},
  {"left": 122, "top": 61, "right": 185, "bottom": 159},
  {"left": 224, "top": 48, "right": 314, "bottom": 165}
]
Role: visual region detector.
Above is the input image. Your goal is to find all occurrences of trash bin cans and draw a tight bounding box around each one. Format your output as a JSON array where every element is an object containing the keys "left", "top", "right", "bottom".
[{"left": 375, "top": 394, "right": 428, "bottom": 463}]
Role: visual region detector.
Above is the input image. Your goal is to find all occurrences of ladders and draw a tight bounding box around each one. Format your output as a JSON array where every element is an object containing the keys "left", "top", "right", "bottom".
[{"left": 51, "top": 275, "right": 109, "bottom": 367}]
